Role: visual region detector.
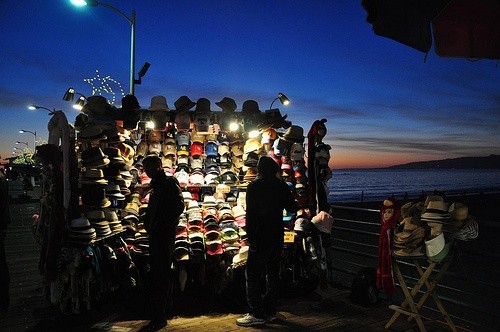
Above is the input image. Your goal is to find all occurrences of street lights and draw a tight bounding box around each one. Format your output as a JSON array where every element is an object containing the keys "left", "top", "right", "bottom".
[
  {"left": 28, "top": 104, "right": 62, "bottom": 146},
  {"left": 71, "top": 0, "right": 151, "bottom": 96},
  {"left": 18, "top": 129, "right": 37, "bottom": 156},
  {"left": 12, "top": 146, "right": 24, "bottom": 157},
  {"left": 16, "top": 141, "right": 29, "bottom": 156}
]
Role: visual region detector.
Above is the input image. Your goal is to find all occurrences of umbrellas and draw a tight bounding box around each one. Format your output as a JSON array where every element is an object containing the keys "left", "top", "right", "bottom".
[{"left": 362, "top": 0, "right": 500, "bottom": 61}]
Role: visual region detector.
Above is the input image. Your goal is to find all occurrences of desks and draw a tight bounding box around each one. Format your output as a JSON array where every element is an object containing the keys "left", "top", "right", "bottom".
[{"left": 384, "top": 251, "right": 461, "bottom": 332}]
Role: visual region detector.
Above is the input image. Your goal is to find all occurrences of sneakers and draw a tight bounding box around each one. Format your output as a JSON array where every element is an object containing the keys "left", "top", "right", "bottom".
[{"left": 235, "top": 313, "right": 267, "bottom": 327}]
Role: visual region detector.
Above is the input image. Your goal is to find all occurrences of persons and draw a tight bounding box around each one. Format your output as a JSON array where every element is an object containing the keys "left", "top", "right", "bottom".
[
  {"left": 0, "top": 179, "right": 11, "bottom": 313},
  {"left": 382, "top": 198, "right": 398, "bottom": 223},
  {"left": 140, "top": 155, "right": 184, "bottom": 332},
  {"left": 235, "top": 155, "right": 299, "bottom": 326}
]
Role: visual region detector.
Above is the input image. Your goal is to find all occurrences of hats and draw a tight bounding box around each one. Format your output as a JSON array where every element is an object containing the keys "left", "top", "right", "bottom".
[
  {"left": 146, "top": 96, "right": 171, "bottom": 112},
  {"left": 215, "top": 97, "right": 237, "bottom": 113},
  {"left": 174, "top": 96, "right": 197, "bottom": 115},
  {"left": 66, "top": 112, "right": 316, "bottom": 270},
  {"left": 241, "top": 100, "right": 261, "bottom": 114},
  {"left": 120, "top": 94, "right": 141, "bottom": 109},
  {"left": 86, "top": 95, "right": 107, "bottom": 116},
  {"left": 311, "top": 211, "right": 335, "bottom": 234},
  {"left": 391, "top": 195, "right": 479, "bottom": 262},
  {"left": 196, "top": 98, "right": 210, "bottom": 112}
]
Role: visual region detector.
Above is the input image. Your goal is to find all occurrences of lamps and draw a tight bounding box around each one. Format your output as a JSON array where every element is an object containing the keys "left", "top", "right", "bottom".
[
  {"left": 62, "top": 87, "right": 87, "bottom": 101},
  {"left": 73, "top": 96, "right": 85, "bottom": 110},
  {"left": 269, "top": 93, "right": 289, "bottom": 109}
]
